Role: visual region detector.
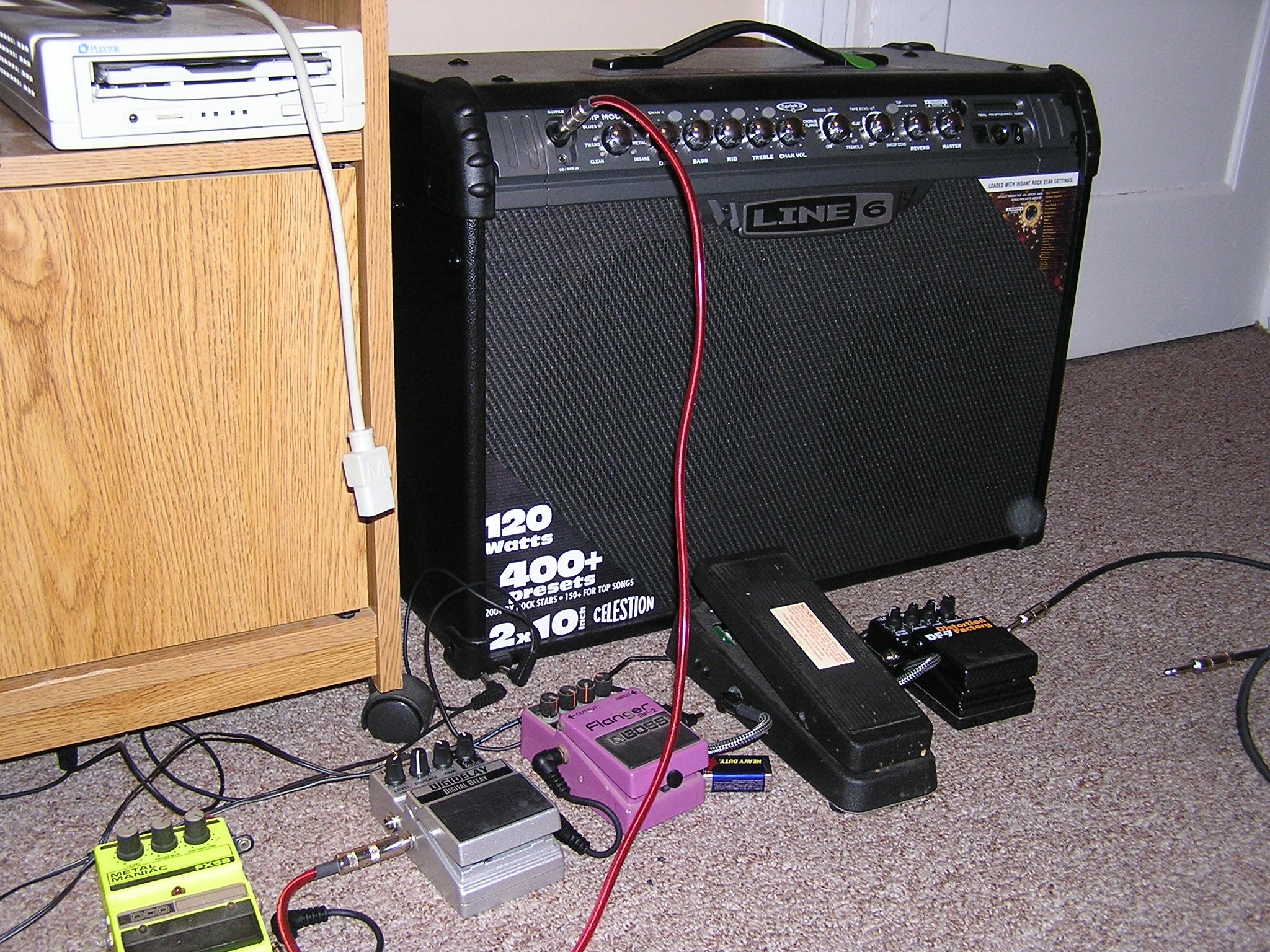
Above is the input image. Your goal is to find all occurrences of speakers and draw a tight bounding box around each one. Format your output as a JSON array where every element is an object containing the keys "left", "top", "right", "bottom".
[{"left": 388, "top": 44, "right": 1099, "bottom": 677}]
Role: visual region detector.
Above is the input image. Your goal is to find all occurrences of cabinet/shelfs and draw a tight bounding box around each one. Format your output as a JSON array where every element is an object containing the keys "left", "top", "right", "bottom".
[{"left": 0, "top": 0, "right": 403, "bottom": 761}]
